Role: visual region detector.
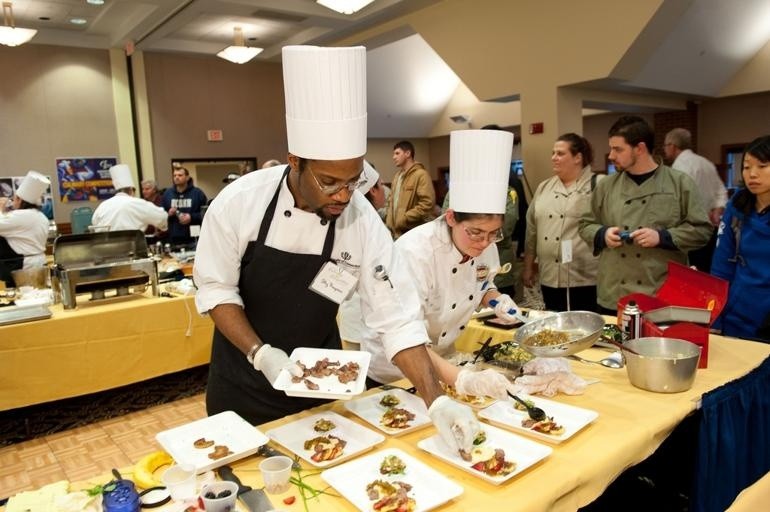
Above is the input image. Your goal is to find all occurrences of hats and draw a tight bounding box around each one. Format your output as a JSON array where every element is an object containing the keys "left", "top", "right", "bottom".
[
  {"left": 447, "top": 128, "right": 515, "bottom": 214},
  {"left": 15, "top": 170, "right": 51, "bottom": 205},
  {"left": 108, "top": 162, "right": 136, "bottom": 192},
  {"left": 281, "top": 41, "right": 371, "bottom": 162}
]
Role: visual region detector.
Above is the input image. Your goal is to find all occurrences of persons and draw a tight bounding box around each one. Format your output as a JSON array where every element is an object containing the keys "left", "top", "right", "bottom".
[
  {"left": 522, "top": 131, "right": 607, "bottom": 313},
  {"left": 1, "top": 192, "right": 50, "bottom": 308},
  {"left": 353, "top": 210, "right": 521, "bottom": 399},
  {"left": 442, "top": 123, "right": 519, "bottom": 303},
  {"left": 158, "top": 167, "right": 208, "bottom": 253},
  {"left": 385, "top": 140, "right": 437, "bottom": 241},
  {"left": 661, "top": 128, "right": 729, "bottom": 269},
  {"left": 340, "top": 175, "right": 394, "bottom": 350},
  {"left": 575, "top": 114, "right": 715, "bottom": 317},
  {"left": 139, "top": 179, "right": 164, "bottom": 237},
  {"left": 189, "top": 155, "right": 483, "bottom": 458},
  {"left": 89, "top": 187, "right": 169, "bottom": 236},
  {"left": 696, "top": 134, "right": 770, "bottom": 511}
]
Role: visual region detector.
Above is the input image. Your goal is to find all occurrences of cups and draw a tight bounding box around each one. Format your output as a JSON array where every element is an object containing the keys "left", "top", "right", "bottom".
[
  {"left": 259, "top": 456, "right": 294, "bottom": 492},
  {"left": 200, "top": 481, "right": 238, "bottom": 512},
  {"left": 160, "top": 463, "right": 198, "bottom": 504}
]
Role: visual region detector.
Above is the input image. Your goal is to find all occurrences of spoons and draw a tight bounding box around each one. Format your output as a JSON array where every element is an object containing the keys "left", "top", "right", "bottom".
[
  {"left": 504, "top": 389, "right": 545, "bottom": 422},
  {"left": 572, "top": 355, "right": 621, "bottom": 369}
]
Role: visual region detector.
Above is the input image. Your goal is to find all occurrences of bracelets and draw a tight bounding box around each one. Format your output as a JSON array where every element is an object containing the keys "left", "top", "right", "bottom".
[{"left": 245, "top": 341, "right": 259, "bottom": 367}]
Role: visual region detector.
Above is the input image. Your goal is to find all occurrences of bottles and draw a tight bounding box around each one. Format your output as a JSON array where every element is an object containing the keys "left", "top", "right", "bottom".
[
  {"left": 623, "top": 301, "right": 642, "bottom": 343},
  {"left": 155, "top": 240, "right": 186, "bottom": 266},
  {"left": 100, "top": 479, "right": 142, "bottom": 511}
]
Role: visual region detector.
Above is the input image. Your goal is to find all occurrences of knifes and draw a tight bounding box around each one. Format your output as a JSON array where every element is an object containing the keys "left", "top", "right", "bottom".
[
  {"left": 264, "top": 445, "right": 302, "bottom": 469},
  {"left": 218, "top": 467, "right": 274, "bottom": 511}
]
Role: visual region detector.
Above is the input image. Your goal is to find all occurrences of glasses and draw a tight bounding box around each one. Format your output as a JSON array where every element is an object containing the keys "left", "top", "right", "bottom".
[
  {"left": 460, "top": 221, "right": 505, "bottom": 244},
  {"left": 300, "top": 160, "right": 369, "bottom": 196}
]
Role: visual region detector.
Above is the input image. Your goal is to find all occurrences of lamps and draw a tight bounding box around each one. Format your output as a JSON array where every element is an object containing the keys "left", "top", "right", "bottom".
[
  {"left": 215, "top": 22, "right": 267, "bottom": 68},
  {"left": 0, "top": 1, "right": 39, "bottom": 51}
]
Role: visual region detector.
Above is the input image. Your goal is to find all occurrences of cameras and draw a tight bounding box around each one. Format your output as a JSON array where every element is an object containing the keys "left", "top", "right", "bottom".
[{"left": 619, "top": 233, "right": 633, "bottom": 245}]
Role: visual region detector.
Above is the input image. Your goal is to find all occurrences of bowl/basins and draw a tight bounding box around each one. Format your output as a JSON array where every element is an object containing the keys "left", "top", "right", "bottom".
[
  {"left": 621, "top": 337, "right": 701, "bottom": 393},
  {"left": 11, "top": 267, "right": 50, "bottom": 292},
  {"left": 489, "top": 360, "right": 521, "bottom": 381}
]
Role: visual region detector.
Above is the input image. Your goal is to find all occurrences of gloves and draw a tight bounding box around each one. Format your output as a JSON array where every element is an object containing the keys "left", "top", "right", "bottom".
[
  {"left": 426, "top": 392, "right": 479, "bottom": 457},
  {"left": 492, "top": 292, "right": 519, "bottom": 326},
  {"left": 454, "top": 368, "right": 518, "bottom": 405},
  {"left": 249, "top": 342, "right": 304, "bottom": 396}
]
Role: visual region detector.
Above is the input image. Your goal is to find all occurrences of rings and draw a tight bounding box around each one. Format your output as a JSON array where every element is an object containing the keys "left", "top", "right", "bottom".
[{"left": 641, "top": 238, "right": 646, "bottom": 243}]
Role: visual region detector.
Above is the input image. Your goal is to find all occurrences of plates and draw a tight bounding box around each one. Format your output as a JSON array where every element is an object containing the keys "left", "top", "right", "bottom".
[
  {"left": 273, "top": 346, "right": 372, "bottom": 399},
  {"left": 419, "top": 421, "right": 552, "bottom": 488},
  {"left": 267, "top": 410, "right": 385, "bottom": 469},
  {"left": 319, "top": 447, "right": 463, "bottom": 512},
  {"left": 482, "top": 395, "right": 599, "bottom": 444},
  {"left": 158, "top": 407, "right": 269, "bottom": 474},
  {"left": 346, "top": 387, "right": 434, "bottom": 438}
]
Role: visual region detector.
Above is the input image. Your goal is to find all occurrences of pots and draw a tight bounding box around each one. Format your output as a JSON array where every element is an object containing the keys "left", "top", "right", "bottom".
[{"left": 487, "top": 295, "right": 605, "bottom": 358}]
